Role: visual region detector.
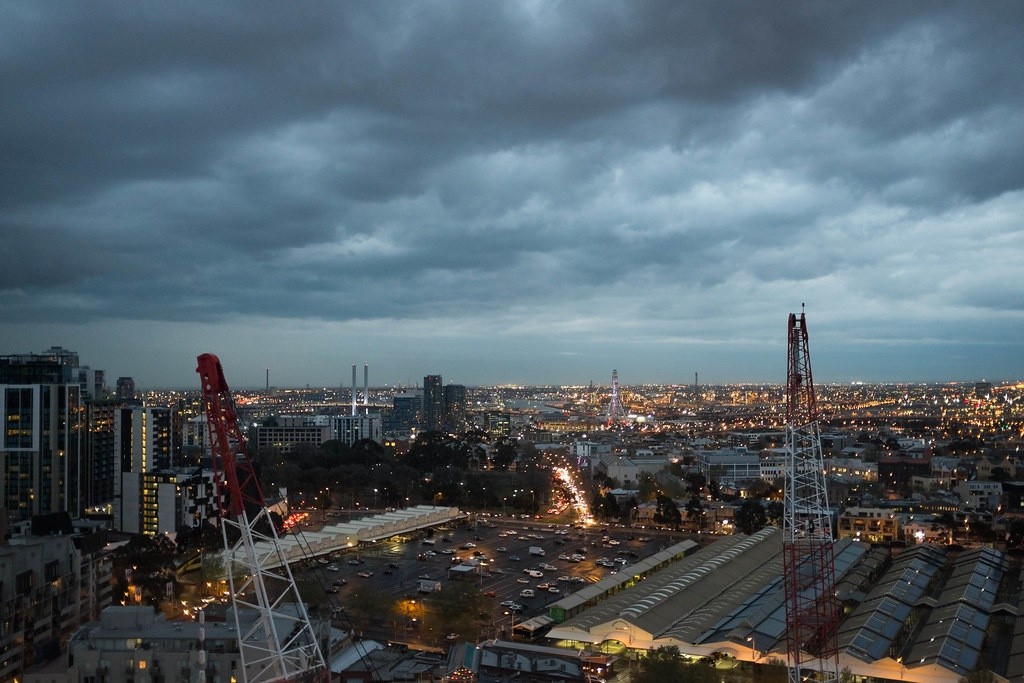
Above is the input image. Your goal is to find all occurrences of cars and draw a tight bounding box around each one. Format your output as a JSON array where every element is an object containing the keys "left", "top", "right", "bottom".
[{"left": 314, "top": 512, "right": 655, "bottom": 615}]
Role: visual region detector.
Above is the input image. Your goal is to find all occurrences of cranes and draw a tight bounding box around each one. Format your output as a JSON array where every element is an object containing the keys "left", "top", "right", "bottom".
[
  {"left": 196, "top": 353, "right": 337, "bottom": 683},
  {"left": 782, "top": 311, "right": 846, "bottom": 683}
]
